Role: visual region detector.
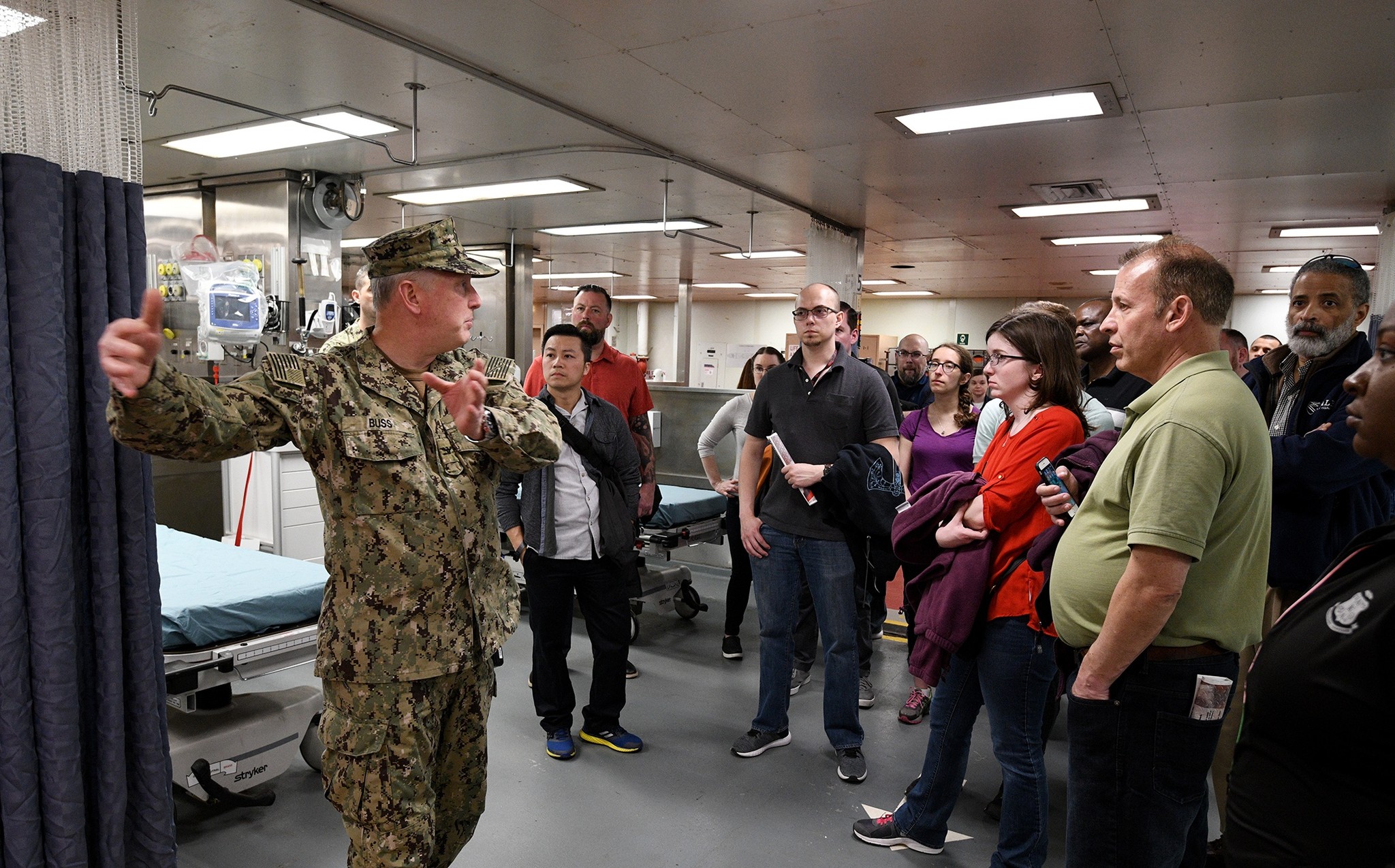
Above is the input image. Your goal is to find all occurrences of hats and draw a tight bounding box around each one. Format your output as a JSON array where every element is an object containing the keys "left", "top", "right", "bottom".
[{"left": 361, "top": 215, "right": 500, "bottom": 280}]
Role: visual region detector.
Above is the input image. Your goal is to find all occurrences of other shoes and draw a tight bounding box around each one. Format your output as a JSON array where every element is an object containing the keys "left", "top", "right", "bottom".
[
  {"left": 625, "top": 660, "right": 638, "bottom": 679},
  {"left": 528, "top": 671, "right": 533, "bottom": 688},
  {"left": 722, "top": 635, "right": 743, "bottom": 659}
]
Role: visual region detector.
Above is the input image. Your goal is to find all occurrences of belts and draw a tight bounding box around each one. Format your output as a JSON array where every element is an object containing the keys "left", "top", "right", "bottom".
[{"left": 1079, "top": 640, "right": 1231, "bottom": 661}]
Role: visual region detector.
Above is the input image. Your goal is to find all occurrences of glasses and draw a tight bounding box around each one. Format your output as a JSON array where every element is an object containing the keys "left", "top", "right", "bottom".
[
  {"left": 1304, "top": 254, "right": 1361, "bottom": 265},
  {"left": 986, "top": 353, "right": 1034, "bottom": 366},
  {"left": 926, "top": 361, "right": 966, "bottom": 373},
  {"left": 792, "top": 306, "right": 838, "bottom": 321},
  {"left": 894, "top": 350, "right": 928, "bottom": 360},
  {"left": 752, "top": 366, "right": 774, "bottom": 374}
]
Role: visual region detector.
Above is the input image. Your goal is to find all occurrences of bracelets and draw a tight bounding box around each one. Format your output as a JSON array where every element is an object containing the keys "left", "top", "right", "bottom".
[{"left": 512, "top": 540, "right": 526, "bottom": 563}]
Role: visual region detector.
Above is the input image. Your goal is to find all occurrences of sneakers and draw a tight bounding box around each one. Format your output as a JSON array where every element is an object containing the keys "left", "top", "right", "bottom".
[
  {"left": 899, "top": 685, "right": 933, "bottom": 725},
  {"left": 834, "top": 746, "right": 868, "bottom": 784},
  {"left": 859, "top": 676, "right": 875, "bottom": 707},
  {"left": 578, "top": 721, "right": 643, "bottom": 753},
  {"left": 853, "top": 812, "right": 944, "bottom": 855},
  {"left": 730, "top": 727, "right": 792, "bottom": 758},
  {"left": 546, "top": 727, "right": 576, "bottom": 760},
  {"left": 790, "top": 668, "right": 812, "bottom": 696}
]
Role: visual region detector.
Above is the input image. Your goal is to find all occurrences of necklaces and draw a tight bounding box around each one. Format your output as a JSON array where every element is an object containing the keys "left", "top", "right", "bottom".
[
  {"left": 811, "top": 346, "right": 837, "bottom": 386},
  {"left": 1002, "top": 410, "right": 1030, "bottom": 448},
  {"left": 931, "top": 401, "right": 957, "bottom": 436}
]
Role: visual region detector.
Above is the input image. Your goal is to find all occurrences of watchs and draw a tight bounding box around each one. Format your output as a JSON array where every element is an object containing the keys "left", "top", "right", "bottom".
[
  {"left": 824, "top": 464, "right": 833, "bottom": 477},
  {"left": 480, "top": 407, "right": 496, "bottom": 440}
]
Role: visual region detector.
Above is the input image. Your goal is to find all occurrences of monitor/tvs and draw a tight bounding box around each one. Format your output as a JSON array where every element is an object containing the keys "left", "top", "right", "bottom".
[{"left": 210, "top": 293, "right": 259, "bottom": 329}]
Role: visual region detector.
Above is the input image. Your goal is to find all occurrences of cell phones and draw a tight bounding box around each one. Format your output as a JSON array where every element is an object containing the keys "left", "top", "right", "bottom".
[{"left": 1035, "top": 456, "right": 1078, "bottom": 520}]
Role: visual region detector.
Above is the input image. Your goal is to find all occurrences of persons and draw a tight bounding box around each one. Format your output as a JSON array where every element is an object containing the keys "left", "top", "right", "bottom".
[
  {"left": 698, "top": 345, "right": 785, "bottom": 658},
  {"left": 100, "top": 218, "right": 557, "bottom": 868},
  {"left": 523, "top": 284, "right": 656, "bottom": 688},
  {"left": 784, "top": 296, "right": 1151, "bottom": 824},
  {"left": 1050, "top": 240, "right": 1273, "bottom": 868},
  {"left": 851, "top": 308, "right": 1082, "bottom": 868},
  {"left": 729, "top": 284, "right": 898, "bottom": 783},
  {"left": 1203, "top": 255, "right": 1395, "bottom": 868},
  {"left": 496, "top": 325, "right": 643, "bottom": 761},
  {"left": 317, "top": 261, "right": 379, "bottom": 353}
]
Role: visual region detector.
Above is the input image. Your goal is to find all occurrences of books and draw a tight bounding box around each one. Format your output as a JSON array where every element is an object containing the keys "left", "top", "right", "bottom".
[{"left": 1189, "top": 674, "right": 1233, "bottom": 721}]
